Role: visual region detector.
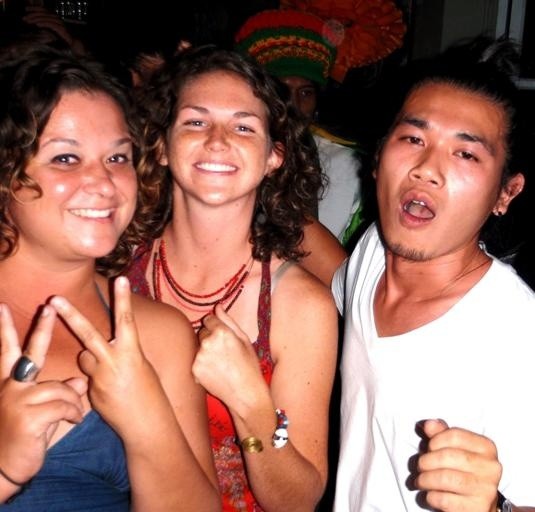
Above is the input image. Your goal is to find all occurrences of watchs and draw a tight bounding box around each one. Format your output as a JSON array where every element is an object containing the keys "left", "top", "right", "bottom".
[{"left": 496, "top": 490, "right": 512, "bottom": 512}]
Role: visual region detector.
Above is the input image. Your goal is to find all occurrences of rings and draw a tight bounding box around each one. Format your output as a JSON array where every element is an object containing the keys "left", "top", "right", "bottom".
[{"left": 14, "top": 355, "right": 42, "bottom": 383}]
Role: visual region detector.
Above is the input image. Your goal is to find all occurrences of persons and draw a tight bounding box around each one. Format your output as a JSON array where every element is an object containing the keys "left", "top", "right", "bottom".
[
  {"left": 115, "top": 38, "right": 339, "bottom": 512},
  {"left": 0, "top": 24, "right": 224, "bottom": 512},
  {"left": 233, "top": 8, "right": 366, "bottom": 247},
  {"left": 290, "top": 42, "right": 535, "bottom": 512}
]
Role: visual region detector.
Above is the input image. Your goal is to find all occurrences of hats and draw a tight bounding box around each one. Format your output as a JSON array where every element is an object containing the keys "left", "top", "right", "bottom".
[{"left": 232, "top": 8, "right": 339, "bottom": 91}]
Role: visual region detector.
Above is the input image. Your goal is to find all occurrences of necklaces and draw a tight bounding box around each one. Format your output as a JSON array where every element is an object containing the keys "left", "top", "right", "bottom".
[{"left": 146, "top": 237, "right": 256, "bottom": 337}]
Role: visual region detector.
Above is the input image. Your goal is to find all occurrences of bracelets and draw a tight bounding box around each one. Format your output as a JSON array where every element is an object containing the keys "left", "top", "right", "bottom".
[
  {"left": 232, "top": 408, "right": 292, "bottom": 453},
  {"left": 0, "top": 467, "right": 30, "bottom": 487}
]
